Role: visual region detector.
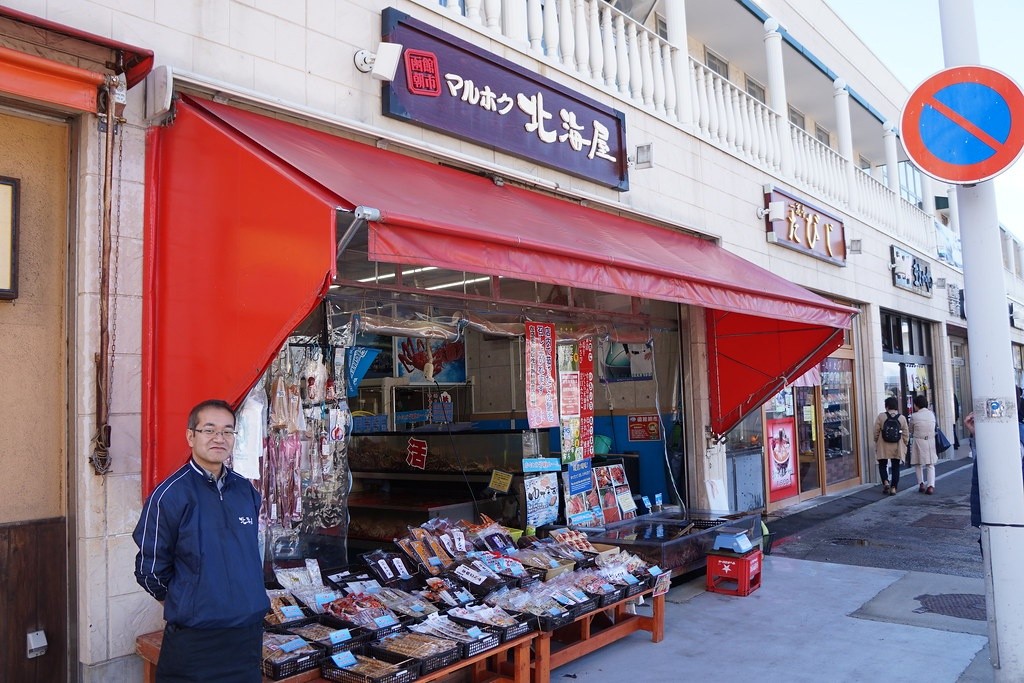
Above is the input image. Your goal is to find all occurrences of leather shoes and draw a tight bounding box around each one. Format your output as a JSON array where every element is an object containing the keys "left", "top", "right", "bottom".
[
  {"left": 925, "top": 487, "right": 934, "bottom": 495},
  {"left": 919, "top": 483, "right": 925, "bottom": 492}
]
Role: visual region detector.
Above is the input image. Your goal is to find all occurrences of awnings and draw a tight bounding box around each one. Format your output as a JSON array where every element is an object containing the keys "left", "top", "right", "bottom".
[{"left": 140, "top": 93, "right": 861, "bottom": 523}]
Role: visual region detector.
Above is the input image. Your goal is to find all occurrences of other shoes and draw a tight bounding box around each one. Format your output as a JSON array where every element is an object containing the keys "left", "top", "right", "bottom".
[
  {"left": 890, "top": 486, "right": 896, "bottom": 495},
  {"left": 883, "top": 484, "right": 889, "bottom": 493}
]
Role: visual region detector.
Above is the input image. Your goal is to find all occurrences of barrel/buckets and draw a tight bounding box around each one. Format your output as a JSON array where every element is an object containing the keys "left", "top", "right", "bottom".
[{"left": 593, "top": 435, "right": 613, "bottom": 458}]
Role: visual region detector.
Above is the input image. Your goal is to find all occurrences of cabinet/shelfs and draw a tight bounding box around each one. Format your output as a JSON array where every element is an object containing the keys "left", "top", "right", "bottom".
[{"left": 799, "top": 358, "right": 853, "bottom": 462}]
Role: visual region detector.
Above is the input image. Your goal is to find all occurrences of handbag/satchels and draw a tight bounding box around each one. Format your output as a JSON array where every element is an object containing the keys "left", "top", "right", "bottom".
[{"left": 935, "top": 426, "right": 951, "bottom": 454}]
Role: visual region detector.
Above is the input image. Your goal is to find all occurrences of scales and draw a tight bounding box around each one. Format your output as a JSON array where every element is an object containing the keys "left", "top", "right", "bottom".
[{"left": 713, "top": 526, "right": 753, "bottom": 554}]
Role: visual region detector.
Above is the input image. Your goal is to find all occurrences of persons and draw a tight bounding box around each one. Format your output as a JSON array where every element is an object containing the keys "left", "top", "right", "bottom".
[
  {"left": 963, "top": 385, "right": 1023, "bottom": 560},
  {"left": 132, "top": 399, "right": 271, "bottom": 683},
  {"left": 909, "top": 395, "right": 938, "bottom": 495},
  {"left": 873, "top": 397, "right": 909, "bottom": 495},
  {"left": 953, "top": 393, "right": 960, "bottom": 450}
]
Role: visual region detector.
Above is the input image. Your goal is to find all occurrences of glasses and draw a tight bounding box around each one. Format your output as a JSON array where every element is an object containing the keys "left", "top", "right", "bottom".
[{"left": 187, "top": 427, "right": 238, "bottom": 439}]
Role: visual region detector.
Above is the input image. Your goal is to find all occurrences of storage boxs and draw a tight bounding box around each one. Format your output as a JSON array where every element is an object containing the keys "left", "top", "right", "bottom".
[
  {"left": 706, "top": 550, "right": 762, "bottom": 597},
  {"left": 250, "top": 526, "right": 666, "bottom": 683}
]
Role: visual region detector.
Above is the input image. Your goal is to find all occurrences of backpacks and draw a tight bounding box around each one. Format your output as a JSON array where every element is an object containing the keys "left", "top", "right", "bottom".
[{"left": 881, "top": 412, "right": 902, "bottom": 443}]
